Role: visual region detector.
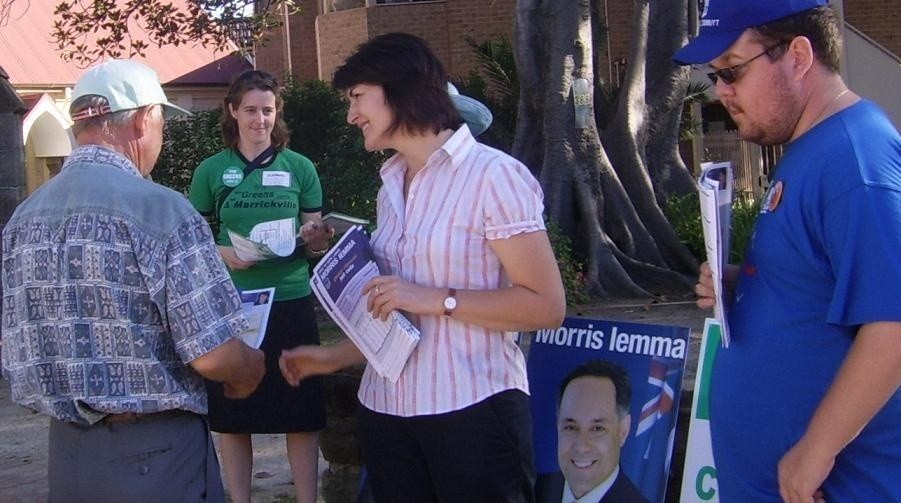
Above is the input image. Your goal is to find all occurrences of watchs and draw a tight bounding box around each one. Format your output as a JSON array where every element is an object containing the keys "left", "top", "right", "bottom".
[{"left": 444, "top": 286, "right": 459, "bottom": 318}]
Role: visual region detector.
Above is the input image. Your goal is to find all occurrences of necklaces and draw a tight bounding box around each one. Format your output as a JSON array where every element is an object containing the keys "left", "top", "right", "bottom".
[{"left": 832, "top": 88, "right": 851, "bottom": 102}]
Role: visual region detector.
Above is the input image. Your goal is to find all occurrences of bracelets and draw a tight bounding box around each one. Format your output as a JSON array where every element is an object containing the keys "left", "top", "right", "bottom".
[{"left": 310, "top": 247, "right": 329, "bottom": 255}]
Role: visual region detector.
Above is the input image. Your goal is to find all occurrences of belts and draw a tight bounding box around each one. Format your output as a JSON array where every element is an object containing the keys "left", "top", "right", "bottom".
[{"left": 101, "top": 408, "right": 189, "bottom": 422}]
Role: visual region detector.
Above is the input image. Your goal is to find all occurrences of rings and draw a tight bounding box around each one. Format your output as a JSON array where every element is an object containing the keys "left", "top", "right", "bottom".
[{"left": 375, "top": 283, "right": 384, "bottom": 295}]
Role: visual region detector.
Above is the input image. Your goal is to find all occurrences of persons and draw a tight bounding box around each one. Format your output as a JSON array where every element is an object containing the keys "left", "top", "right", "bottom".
[
  {"left": 719, "top": 172, "right": 726, "bottom": 190},
  {"left": 534, "top": 357, "right": 649, "bottom": 503},
  {"left": 280, "top": 28, "right": 570, "bottom": 503},
  {"left": 187, "top": 66, "right": 337, "bottom": 502},
  {"left": 0, "top": 58, "right": 268, "bottom": 503},
  {"left": 671, "top": 0, "right": 901, "bottom": 502},
  {"left": 255, "top": 291, "right": 269, "bottom": 305}
]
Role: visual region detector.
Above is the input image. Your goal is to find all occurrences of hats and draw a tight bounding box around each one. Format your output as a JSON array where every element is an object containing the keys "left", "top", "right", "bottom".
[
  {"left": 68, "top": 59, "right": 193, "bottom": 120},
  {"left": 671, "top": 0, "right": 829, "bottom": 66},
  {"left": 447, "top": 82, "right": 492, "bottom": 137}
]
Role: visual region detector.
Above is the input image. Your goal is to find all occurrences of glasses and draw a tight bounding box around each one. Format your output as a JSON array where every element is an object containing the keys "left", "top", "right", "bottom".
[
  {"left": 236, "top": 69, "right": 276, "bottom": 84},
  {"left": 706, "top": 40, "right": 793, "bottom": 84}
]
